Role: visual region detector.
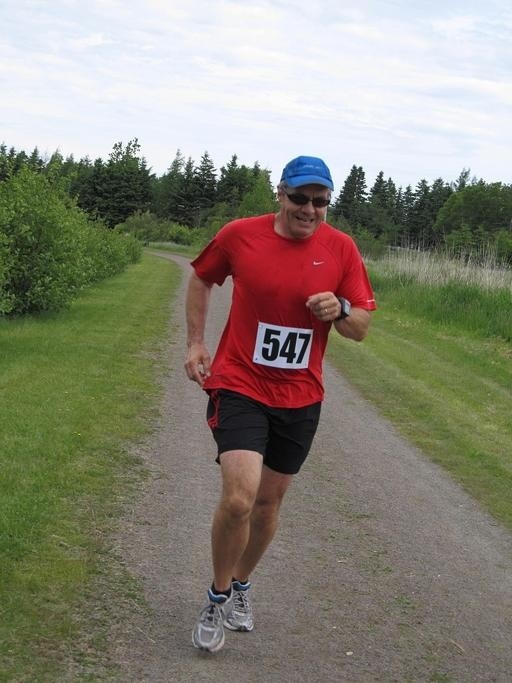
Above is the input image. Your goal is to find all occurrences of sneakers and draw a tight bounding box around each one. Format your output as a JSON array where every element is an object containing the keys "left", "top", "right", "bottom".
[
  {"left": 224, "top": 578, "right": 255, "bottom": 632},
  {"left": 191, "top": 582, "right": 235, "bottom": 654}
]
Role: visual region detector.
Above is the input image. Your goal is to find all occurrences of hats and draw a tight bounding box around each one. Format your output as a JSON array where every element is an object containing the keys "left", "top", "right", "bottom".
[{"left": 280, "top": 156, "right": 334, "bottom": 191}]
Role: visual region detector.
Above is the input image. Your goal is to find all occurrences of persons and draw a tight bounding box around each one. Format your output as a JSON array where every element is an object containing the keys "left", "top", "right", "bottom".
[{"left": 184, "top": 155, "right": 378, "bottom": 652}]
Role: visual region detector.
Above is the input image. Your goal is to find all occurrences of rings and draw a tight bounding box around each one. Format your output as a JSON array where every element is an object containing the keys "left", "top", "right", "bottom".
[{"left": 323, "top": 307, "right": 330, "bottom": 313}]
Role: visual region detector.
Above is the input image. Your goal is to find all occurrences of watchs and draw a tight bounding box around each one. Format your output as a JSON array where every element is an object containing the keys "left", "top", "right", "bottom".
[{"left": 334, "top": 296, "right": 351, "bottom": 321}]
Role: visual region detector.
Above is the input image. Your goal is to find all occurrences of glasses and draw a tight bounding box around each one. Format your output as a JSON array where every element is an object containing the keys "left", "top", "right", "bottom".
[{"left": 280, "top": 184, "right": 332, "bottom": 209}]
